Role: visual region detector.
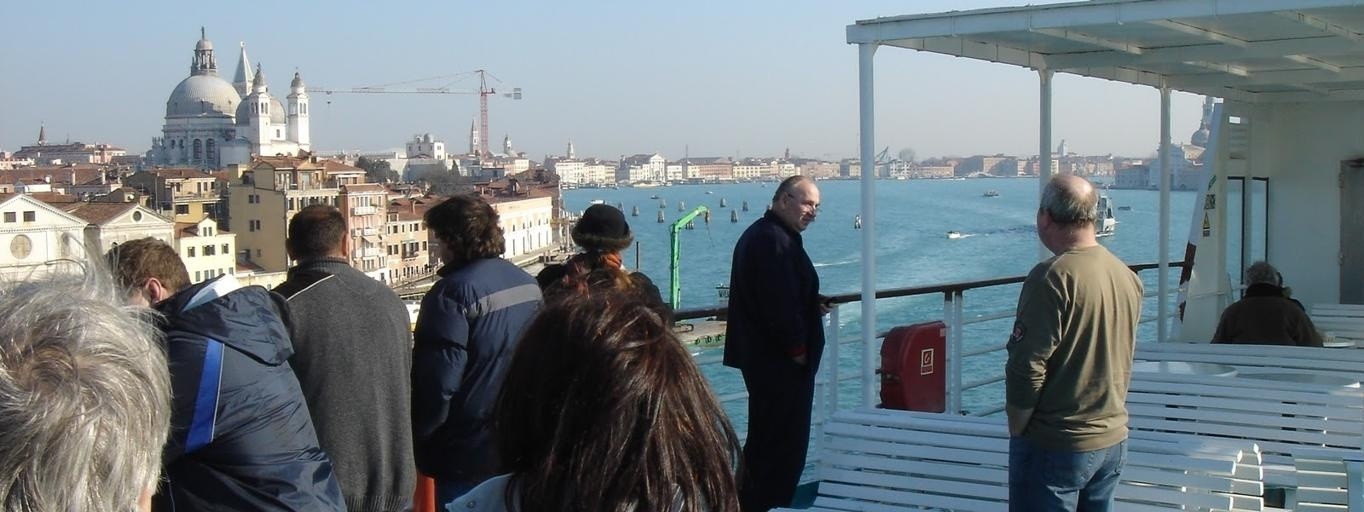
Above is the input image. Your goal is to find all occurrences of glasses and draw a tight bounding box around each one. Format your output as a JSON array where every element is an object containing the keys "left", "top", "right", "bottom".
[{"left": 788, "top": 194, "right": 823, "bottom": 216}]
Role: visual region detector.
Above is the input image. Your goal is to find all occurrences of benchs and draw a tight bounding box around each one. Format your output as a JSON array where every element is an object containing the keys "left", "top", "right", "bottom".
[{"left": 764, "top": 305, "right": 1363, "bottom": 512}]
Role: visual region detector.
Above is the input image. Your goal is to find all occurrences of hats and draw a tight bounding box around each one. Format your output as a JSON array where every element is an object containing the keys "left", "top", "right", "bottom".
[{"left": 571, "top": 203, "right": 634, "bottom": 250}]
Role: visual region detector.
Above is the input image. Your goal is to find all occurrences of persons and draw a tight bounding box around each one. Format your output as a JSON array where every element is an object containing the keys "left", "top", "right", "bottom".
[
  {"left": 722, "top": 176, "right": 842, "bottom": 511},
  {"left": 1006, "top": 172, "right": 1144, "bottom": 511},
  {"left": 1210, "top": 260, "right": 1325, "bottom": 348},
  {"left": 536, "top": 205, "right": 664, "bottom": 319},
  {"left": 0, "top": 232, "right": 170, "bottom": 511},
  {"left": 265, "top": 204, "right": 418, "bottom": 512},
  {"left": 441, "top": 289, "right": 748, "bottom": 512},
  {"left": 410, "top": 193, "right": 544, "bottom": 511},
  {"left": 103, "top": 236, "right": 348, "bottom": 511}
]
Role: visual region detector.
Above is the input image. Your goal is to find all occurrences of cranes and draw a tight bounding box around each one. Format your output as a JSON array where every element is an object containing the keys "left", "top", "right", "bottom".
[{"left": 307, "top": 70, "right": 522, "bottom": 156}]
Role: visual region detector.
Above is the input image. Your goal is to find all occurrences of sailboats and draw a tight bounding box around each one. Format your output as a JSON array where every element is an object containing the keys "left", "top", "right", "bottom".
[{"left": 854, "top": 214, "right": 861, "bottom": 229}]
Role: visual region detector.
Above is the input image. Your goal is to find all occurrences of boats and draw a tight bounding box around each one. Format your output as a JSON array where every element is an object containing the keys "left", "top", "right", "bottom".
[
  {"left": 947, "top": 232, "right": 960, "bottom": 239},
  {"left": 985, "top": 191, "right": 999, "bottom": 197},
  {"left": 1096, "top": 194, "right": 1114, "bottom": 238},
  {"left": 589, "top": 200, "right": 607, "bottom": 204},
  {"left": 1118, "top": 206, "right": 1130, "bottom": 211}
]
